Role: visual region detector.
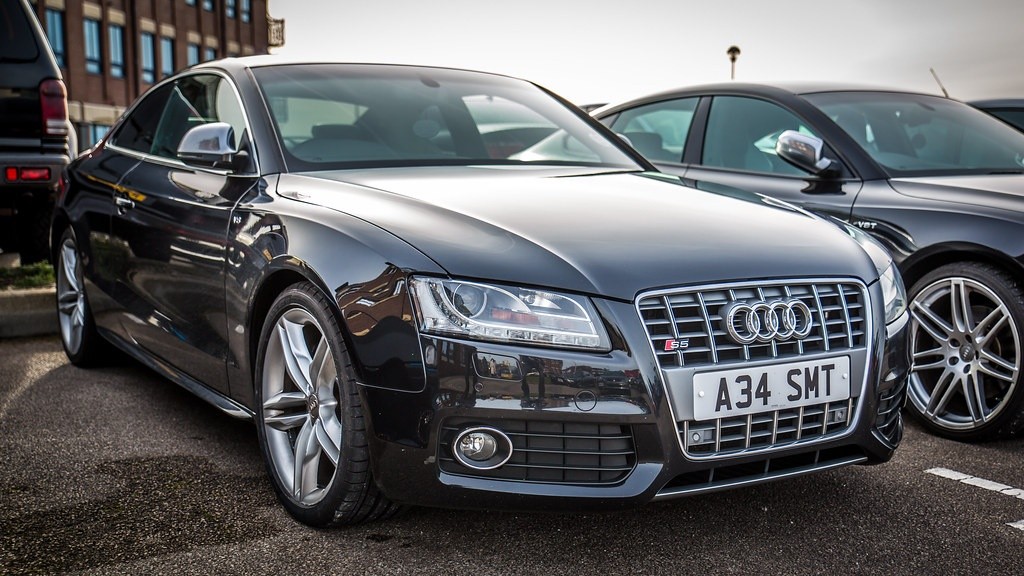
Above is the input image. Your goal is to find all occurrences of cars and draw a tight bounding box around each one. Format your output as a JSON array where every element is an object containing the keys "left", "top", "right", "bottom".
[
  {"left": 0, "top": 1, "right": 79, "bottom": 258},
  {"left": 422, "top": 81, "right": 1024, "bottom": 443},
  {"left": 46, "top": 52, "right": 915, "bottom": 531}
]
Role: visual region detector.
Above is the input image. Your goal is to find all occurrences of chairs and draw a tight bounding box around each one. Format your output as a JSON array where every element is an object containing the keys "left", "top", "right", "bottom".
[
  {"left": 373, "top": 96, "right": 441, "bottom": 151},
  {"left": 835, "top": 109, "right": 875, "bottom": 156},
  {"left": 748, "top": 101, "right": 803, "bottom": 175}
]
